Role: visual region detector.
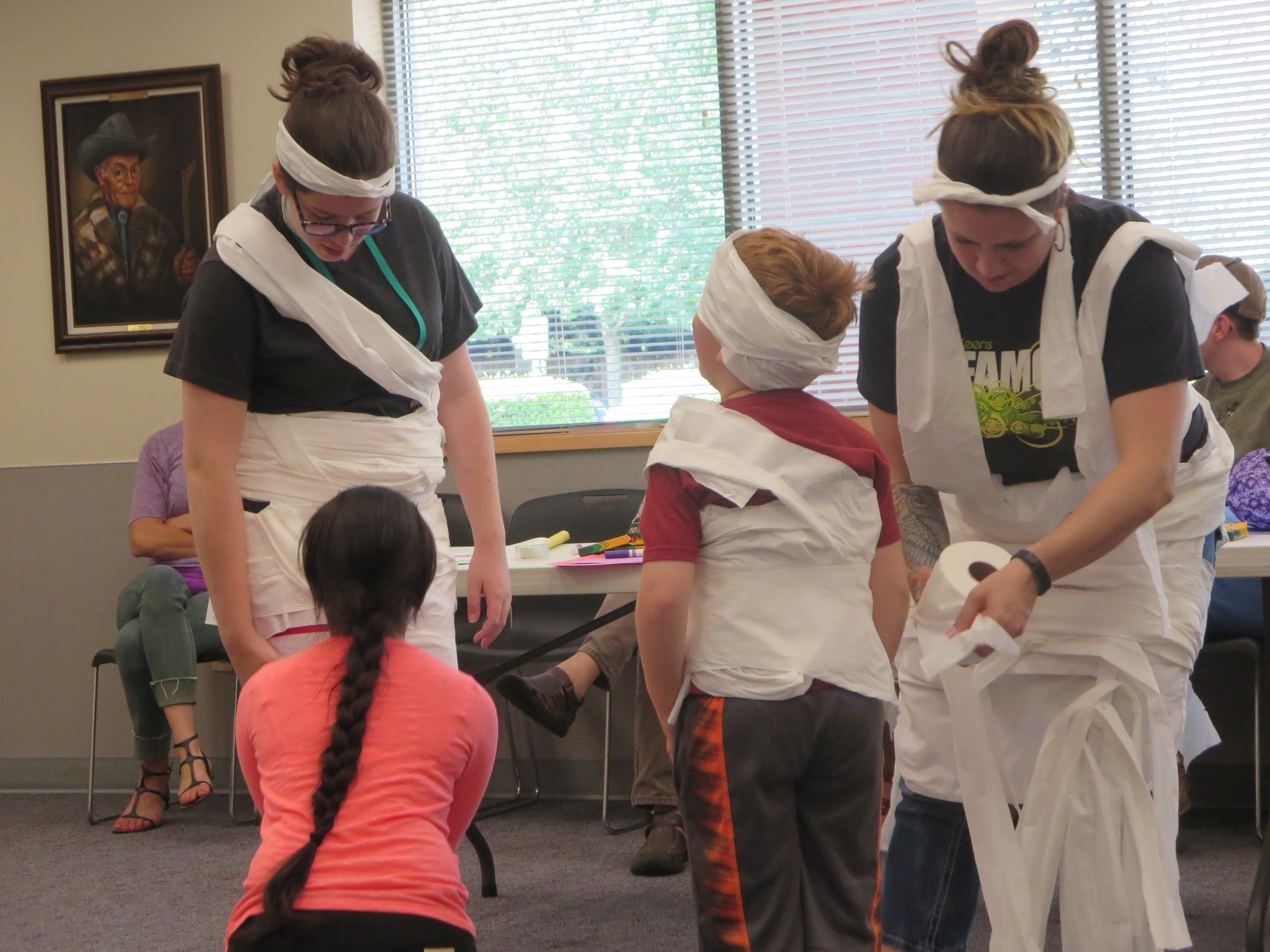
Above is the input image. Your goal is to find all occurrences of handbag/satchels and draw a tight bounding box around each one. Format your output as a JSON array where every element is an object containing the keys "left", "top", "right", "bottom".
[{"left": 1225, "top": 448, "right": 1269, "bottom": 529}]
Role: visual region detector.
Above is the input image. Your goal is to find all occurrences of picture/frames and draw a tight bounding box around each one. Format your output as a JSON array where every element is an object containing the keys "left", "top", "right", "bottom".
[{"left": 36, "top": 60, "right": 230, "bottom": 356}]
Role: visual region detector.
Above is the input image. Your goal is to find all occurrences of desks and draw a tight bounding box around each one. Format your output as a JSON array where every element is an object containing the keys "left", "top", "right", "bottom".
[{"left": 446, "top": 518, "right": 1270, "bottom": 603}]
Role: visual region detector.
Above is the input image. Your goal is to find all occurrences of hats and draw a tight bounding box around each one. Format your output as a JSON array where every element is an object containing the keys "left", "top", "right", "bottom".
[{"left": 1195, "top": 255, "right": 1267, "bottom": 323}]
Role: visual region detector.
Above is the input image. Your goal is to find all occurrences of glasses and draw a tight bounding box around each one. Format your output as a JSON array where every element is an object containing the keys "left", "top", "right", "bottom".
[{"left": 283, "top": 169, "right": 391, "bottom": 236}]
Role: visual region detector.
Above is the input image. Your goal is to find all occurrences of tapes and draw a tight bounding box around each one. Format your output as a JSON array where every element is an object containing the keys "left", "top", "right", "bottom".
[{"left": 516, "top": 544, "right": 548, "bottom": 559}]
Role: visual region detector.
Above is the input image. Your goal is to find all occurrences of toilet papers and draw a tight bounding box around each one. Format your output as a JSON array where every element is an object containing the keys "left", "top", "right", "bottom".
[{"left": 911, "top": 537, "right": 1015, "bottom": 667}]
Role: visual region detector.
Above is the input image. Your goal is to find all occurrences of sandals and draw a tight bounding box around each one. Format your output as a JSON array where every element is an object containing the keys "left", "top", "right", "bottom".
[
  {"left": 112, "top": 763, "right": 173, "bottom": 834},
  {"left": 172, "top": 735, "right": 215, "bottom": 811}
]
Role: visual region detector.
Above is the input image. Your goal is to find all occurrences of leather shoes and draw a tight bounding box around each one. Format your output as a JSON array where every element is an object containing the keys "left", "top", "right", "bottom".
[
  {"left": 496, "top": 666, "right": 585, "bottom": 737},
  {"left": 630, "top": 811, "right": 688, "bottom": 876}
]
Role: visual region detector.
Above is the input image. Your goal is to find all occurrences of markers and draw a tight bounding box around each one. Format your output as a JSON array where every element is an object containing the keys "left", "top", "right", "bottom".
[{"left": 604, "top": 549, "right": 645, "bottom": 559}]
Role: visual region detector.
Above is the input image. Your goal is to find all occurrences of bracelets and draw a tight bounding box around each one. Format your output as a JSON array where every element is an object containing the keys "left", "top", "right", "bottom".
[{"left": 1010, "top": 549, "right": 1052, "bottom": 596}]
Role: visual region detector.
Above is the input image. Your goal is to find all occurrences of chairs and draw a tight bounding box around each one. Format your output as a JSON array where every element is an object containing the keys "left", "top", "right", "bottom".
[
  {"left": 1195, "top": 630, "right": 1264, "bottom": 842},
  {"left": 443, "top": 476, "right": 649, "bottom": 837},
  {"left": 85, "top": 607, "right": 250, "bottom": 831}
]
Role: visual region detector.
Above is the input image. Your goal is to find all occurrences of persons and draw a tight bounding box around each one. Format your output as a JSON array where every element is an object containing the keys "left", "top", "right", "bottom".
[
  {"left": 221, "top": 484, "right": 500, "bottom": 952},
  {"left": 70, "top": 112, "right": 199, "bottom": 322},
  {"left": 496, "top": 494, "right": 692, "bottom": 875},
  {"left": 112, "top": 418, "right": 216, "bottom": 833},
  {"left": 1192, "top": 254, "right": 1270, "bottom": 457},
  {"left": 855, "top": 18, "right": 1234, "bottom": 952},
  {"left": 165, "top": 37, "right": 511, "bottom": 671},
  {"left": 634, "top": 229, "right": 910, "bottom": 952}
]
